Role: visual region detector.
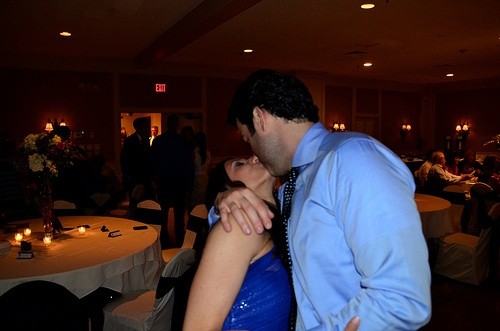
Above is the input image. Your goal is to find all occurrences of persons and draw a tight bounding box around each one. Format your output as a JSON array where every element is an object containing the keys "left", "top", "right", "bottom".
[
  {"left": 208, "top": 68, "right": 432, "bottom": 331},
  {"left": 418, "top": 149, "right": 500, "bottom": 202},
  {"left": 181, "top": 155, "right": 361, "bottom": 331},
  {"left": 120, "top": 113, "right": 213, "bottom": 250}
]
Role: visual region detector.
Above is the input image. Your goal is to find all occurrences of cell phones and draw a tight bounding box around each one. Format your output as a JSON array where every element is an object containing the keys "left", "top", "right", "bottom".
[{"left": 133, "top": 226, "right": 148, "bottom": 230}]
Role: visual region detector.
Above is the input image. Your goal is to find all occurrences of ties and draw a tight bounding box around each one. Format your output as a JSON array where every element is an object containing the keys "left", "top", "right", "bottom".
[{"left": 282, "top": 167, "right": 300, "bottom": 331}]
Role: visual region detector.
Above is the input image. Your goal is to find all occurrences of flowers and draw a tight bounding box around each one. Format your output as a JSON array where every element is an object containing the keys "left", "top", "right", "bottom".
[{"left": 22, "top": 126, "right": 85, "bottom": 177}]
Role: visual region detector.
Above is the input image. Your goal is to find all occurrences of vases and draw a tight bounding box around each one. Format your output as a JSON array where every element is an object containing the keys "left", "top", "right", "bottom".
[{"left": 37, "top": 179, "right": 53, "bottom": 233}]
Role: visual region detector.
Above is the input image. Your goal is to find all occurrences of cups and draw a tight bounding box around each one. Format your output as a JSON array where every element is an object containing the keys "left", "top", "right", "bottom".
[
  {"left": 43, "top": 233, "right": 52, "bottom": 244},
  {"left": 79, "top": 227, "right": 86, "bottom": 234},
  {"left": 15, "top": 233, "right": 23, "bottom": 241},
  {"left": 24, "top": 228, "right": 32, "bottom": 237}
]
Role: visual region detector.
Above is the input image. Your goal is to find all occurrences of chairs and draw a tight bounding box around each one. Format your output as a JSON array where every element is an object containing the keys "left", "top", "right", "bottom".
[
  {"left": 102, "top": 249, "right": 196, "bottom": 331},
  {"left": 53, "top": 200, "right": 77, "bottom": 216},
  {"left": 136, "top": 200, "right": 163, "bottom": 241},
  {"left": 0, "top": 280, "right": 89, "bottom": 331},
  {"left": 467, "top": 183, "right": 494, "bottom": 236},
  {"left": 162, "top": 205, "right": 208, "bottom": 263},
  {"left": 433, "top": 202, "right": 500, "bottom": 288}
]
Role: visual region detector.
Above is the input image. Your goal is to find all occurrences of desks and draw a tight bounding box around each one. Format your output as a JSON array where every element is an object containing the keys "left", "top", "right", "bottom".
[
  {"left": 442, "top": 180, "right": 476, "bottom": 195},
  {"left": 0, "top": 216, "right": 165, "bottom": 299},
  {"left": 415, "top": 193, "right": 456, "bottom": 239}
]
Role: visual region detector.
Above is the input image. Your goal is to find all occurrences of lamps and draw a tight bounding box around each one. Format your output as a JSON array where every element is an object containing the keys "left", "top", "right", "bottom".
[
  {"left": 400, "top": 125, "right": 411, "bottom": 136},
  {"left": 456, "top": 124, "right": 468, "bottom": 137},
  {"left": 45, "top": 118, "right": 66, "bottom": 132},
  {"left": 332, "top": 124, "right": 345, "bottom": 132}
]
